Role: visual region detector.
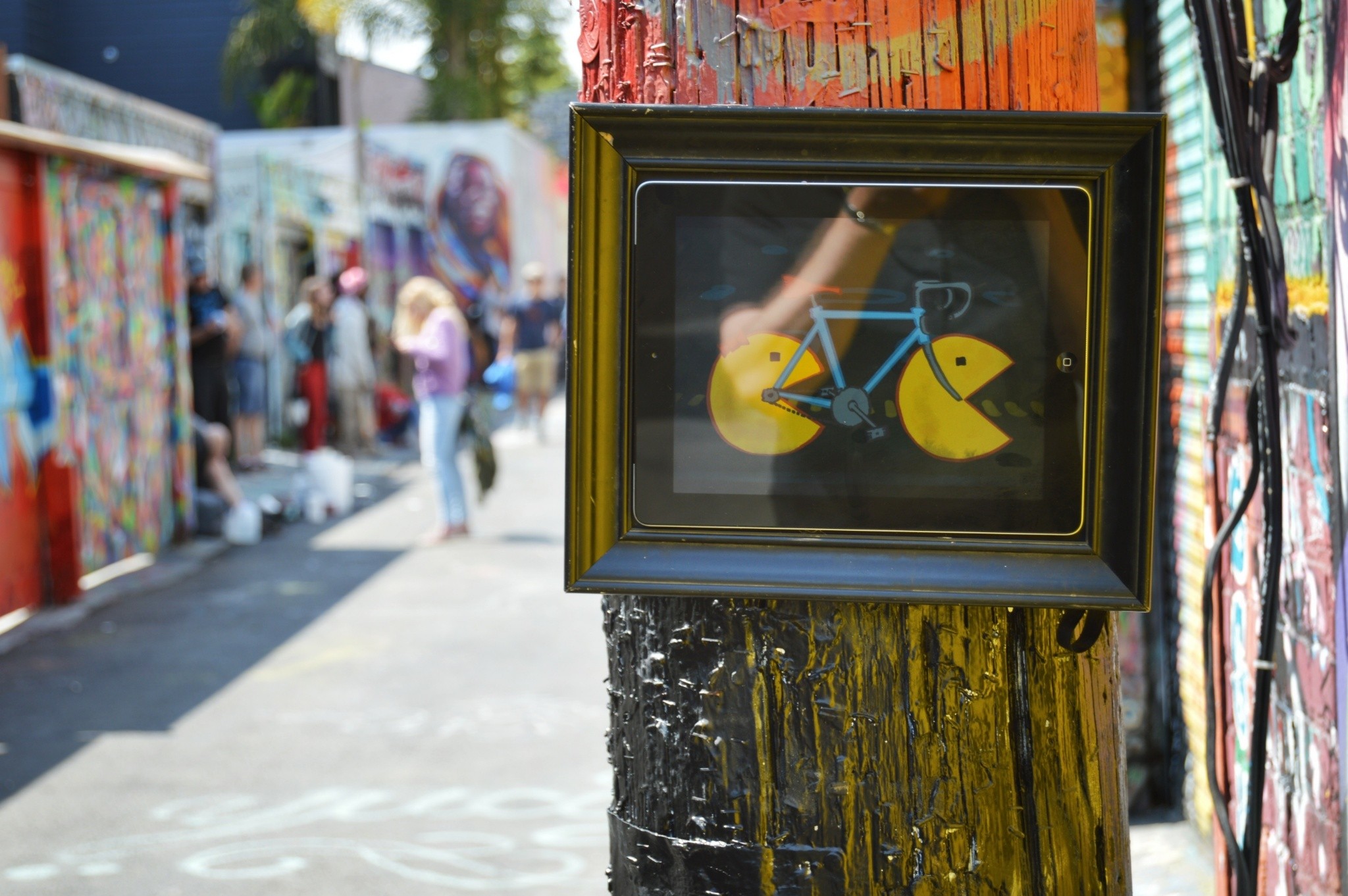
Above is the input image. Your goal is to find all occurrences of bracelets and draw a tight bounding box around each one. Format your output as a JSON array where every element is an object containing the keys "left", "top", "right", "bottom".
[{"left": 840, "top": 199, "right": 899, "bottom": 242}]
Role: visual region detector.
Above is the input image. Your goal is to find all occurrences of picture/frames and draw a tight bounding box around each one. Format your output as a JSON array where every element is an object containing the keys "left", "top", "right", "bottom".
[{"left": 563, "top": 100, "right": 1168, "bottom": 611}]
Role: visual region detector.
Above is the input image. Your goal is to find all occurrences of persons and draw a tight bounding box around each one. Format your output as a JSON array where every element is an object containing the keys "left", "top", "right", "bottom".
[
  {"left": 182, "top": 259, "right": 570, "bottom": 549},
  {"left": 710, "top": 184, "right": 1091, "bottom": 537}
]
